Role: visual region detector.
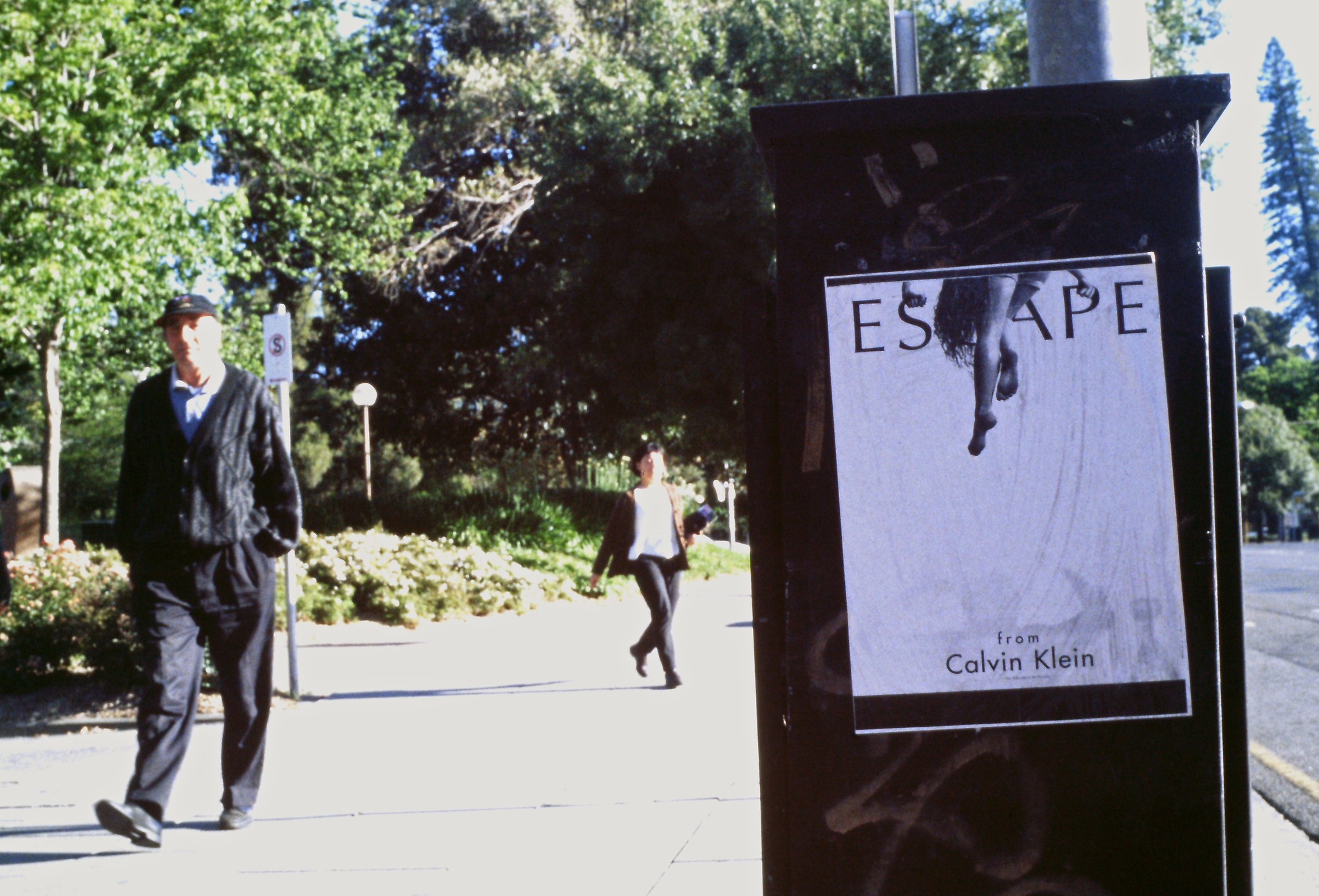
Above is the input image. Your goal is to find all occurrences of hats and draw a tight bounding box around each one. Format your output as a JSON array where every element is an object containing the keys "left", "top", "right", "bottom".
[{"left": 154, "top": 295, "right": 217, "bottom": 324}]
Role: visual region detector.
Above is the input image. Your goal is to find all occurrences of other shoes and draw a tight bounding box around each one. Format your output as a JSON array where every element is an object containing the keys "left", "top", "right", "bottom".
[
  {"left": 630, "top": 644, "right": 647, "bottom": 677},
  {"left": 665, "top": 670, "right": 682, "bottom": 689}
]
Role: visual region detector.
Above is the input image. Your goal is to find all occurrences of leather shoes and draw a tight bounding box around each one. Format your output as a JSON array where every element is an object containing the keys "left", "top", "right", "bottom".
[
  {"left": 219, "top": 806, "right": 252, "bottom": 829},
  {"left": 95, "top": 800, "right": 163, "bottom": 848}
]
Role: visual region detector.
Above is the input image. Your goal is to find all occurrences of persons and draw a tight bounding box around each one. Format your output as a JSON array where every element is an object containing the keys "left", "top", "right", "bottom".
[
  {"left": 94, "top": 292, "right": 302, "bottom": 848},
  {"left": 902, "top": 269, "right": 1095, "bottom": 456},
  {"left": 590, "top": 443, "right": 699, "bottom": 688}
]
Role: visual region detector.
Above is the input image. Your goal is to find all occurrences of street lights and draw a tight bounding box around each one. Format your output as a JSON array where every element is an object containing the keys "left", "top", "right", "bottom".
[{"left": 352, "top": 382, "right": 376, "bottom": 501}]
